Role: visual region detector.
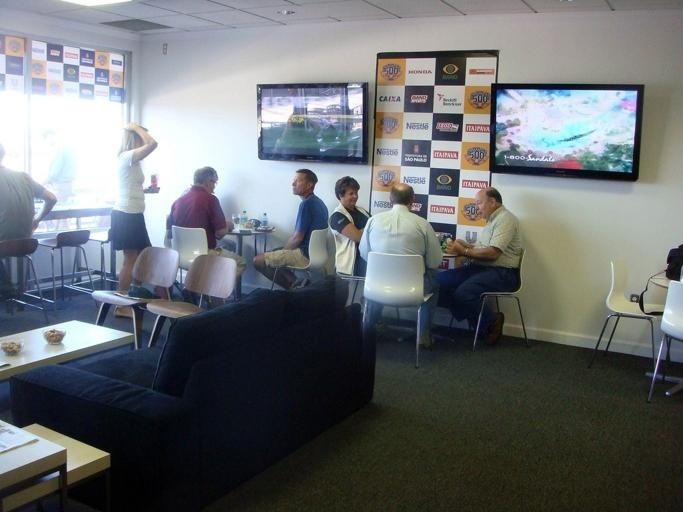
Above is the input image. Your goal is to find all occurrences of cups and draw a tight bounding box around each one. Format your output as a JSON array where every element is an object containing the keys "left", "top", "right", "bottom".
[
  {"left": 232, "top": 213, "right": 241, "bottom": 234},
  {"left": 151, "top": 174, "right": 158, "bottom": 191}
]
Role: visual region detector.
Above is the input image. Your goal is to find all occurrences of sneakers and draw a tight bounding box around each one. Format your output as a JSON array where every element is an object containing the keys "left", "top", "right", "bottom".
[
  {"left": 115, "top": 305, "right": 136, "bottom": 318},
  {"left": 484, "top": 312, "right": 504, "bottom": 346},
  {"left": 479, "top": 314, "right": 487, "bottom": 343}
]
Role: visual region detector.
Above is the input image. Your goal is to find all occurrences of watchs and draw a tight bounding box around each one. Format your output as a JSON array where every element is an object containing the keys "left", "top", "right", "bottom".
[{"left": 464, "top": 248, "right": 468, "bottom": 256}]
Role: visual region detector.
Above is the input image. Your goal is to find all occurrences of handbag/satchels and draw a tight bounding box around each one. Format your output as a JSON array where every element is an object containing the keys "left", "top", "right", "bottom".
[{"left": 666, "top": 245, "right": 683, "bottom": 280}]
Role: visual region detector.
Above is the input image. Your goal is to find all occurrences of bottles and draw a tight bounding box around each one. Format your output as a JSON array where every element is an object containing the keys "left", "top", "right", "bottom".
[
  {"left": 261, "top": 213, "right": 267, "bottom": 226},
  {"left": 443, "top": 233, "right": 452, "bottom": 243},
  {"left": 240, "top": 211, "right": 248, "bottom": 229}
]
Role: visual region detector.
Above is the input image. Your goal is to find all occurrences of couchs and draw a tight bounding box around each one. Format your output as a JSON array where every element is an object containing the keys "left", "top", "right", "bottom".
[{"left": 11, "top": 290, "right": 377, "bottom": 511}]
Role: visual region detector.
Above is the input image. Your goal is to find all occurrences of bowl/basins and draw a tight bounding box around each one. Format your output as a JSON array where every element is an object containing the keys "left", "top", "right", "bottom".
[
  {"left": 42, "top": 328, "right": 66, "bottom": 345},
  {"left": 2, "top": 342, "right": 24, "bottom": 354}
]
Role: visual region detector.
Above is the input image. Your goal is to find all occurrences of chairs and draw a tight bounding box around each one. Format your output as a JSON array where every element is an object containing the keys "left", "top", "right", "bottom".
[
  {"left": 146, "top": 256, "right": 237, "bottom": 347},
  {"left": 328, "top": 226, "right": 365, "bottom": 305},
  {"left": 362, "top": 253, "right": 435, "bottom": 368},
  {"left": 588, "top": 260, "right": 668, "bottom": 378},
  {"left": 647, "top": 280, "right": 683, "bottom": 403},
  {"left": 170, "top": 226, "right": 209, "bottom": 309},
  {"left": 270, "top": 228, "right": 335, "bottom": 291},
  {"left": 448, "top": 248, "right": 526, "bottom": 351},
  {"left": 91, "top": 247, "right": 179, "bottom": 349}
]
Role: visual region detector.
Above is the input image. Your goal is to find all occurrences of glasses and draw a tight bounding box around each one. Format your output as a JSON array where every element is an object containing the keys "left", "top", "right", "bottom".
[{"left": 208, "top": 179, "right": 217, "bottom": 186}]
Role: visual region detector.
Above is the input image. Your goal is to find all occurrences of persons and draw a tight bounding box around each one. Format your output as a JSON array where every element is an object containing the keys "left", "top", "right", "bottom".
[
  {"left": 328, "top": 175, "right": 384, "bottom": 325},
  {"left": 252, "top": 169, "right": 329, "bottom": 290},
  {"left": 358, "top": 183, "right": 443, "bottom": 348},
  {"left": 111, "top": 124, "right": 158, "bottom": 318},
  {"left": 171, "top": 167, "right": 248, "bottom": 304},
  {"left": 436, "top": 187, "right": 522, "bottom": 347},
  {"left": 0, "top": 143, "right": 56, "bottom": 301}
]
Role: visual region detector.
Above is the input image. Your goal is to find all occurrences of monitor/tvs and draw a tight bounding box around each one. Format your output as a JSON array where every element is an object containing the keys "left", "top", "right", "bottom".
[
  {"left": 256, "top": 82, "right": 369, "bottom": 163},
  {"left": 490, "top": 82, "right": 645, "bottom": 182}
]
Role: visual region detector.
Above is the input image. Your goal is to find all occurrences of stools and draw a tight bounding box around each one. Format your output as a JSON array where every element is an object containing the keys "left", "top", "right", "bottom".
[
  {"left": 0, "top": 239, "right": 49, "bottom": 322},
  {"left": 24, "top": 230, "right": 97, "bottom": 311},
  {"left": 71, "top": 225, "right": 115, "bottom": 312}
]
